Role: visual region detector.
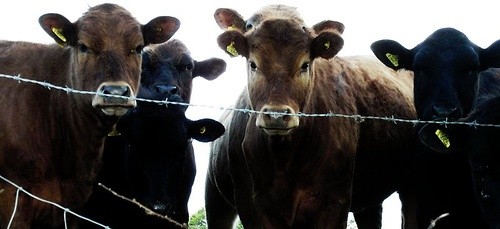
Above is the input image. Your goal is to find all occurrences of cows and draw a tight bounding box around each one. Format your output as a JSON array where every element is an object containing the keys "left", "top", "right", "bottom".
[
  {"left": 0, "top": 4, "right": 180, "bottom": 229},
  {"left": 369, "top": 28, "right": 500, "bottom": 229},
  {"left": 78, "top": 5, "right": 413, "bottom": 227}
]
